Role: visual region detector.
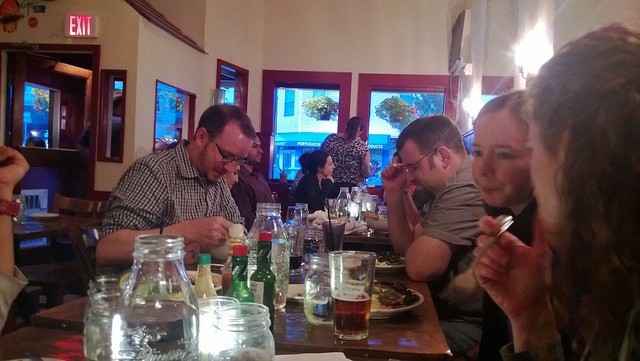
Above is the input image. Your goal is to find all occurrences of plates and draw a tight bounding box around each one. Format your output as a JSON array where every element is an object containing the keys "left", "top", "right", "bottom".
[
  {"left": 368, "top": 279, "right": 425, "bottom": 319},
  {"left": 373, "top": 255, "right": 408, "bottom": 275},
  {"left": 118, "top": 270, "right": 225, "bottom": 295},
  {"left": 288, "top": 284, "right": 306, "bottom": 303},
  {"left": 30, "top": 213, "right": 60, "bottom": 219}
]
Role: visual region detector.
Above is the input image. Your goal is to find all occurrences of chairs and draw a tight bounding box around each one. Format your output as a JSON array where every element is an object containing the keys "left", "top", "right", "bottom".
[
  {"left": 19, "top": 189, "right": 48, "bottom": 266},
  {"left": 67, "top": 223, "right": 101, "bottom": 278},
  {"left": 48, "top": 191, "right": 112, "bottom": 263}
]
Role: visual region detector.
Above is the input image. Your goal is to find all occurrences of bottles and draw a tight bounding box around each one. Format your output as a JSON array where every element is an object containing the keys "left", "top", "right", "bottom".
[
  {"left": 194, "top": 253, "right": 218, "bottom": 296},
  {"left": 248, "top": 202, "right": 290, "bottom": 307},
  {"left": 225, "top": 245, "right": 255, "bottom": 302},
  {"left": 122, "top": 233, "right": 199, "bottom": 360},
  {"left": 352, "top": 186, "right": 362, "bottom": 217},
  {"left": 338, "top": 186, "right": 351, "bottom": 213},
  {"left": 223, "top": 239, "right": 242, "bottom": 290},
  {"left": 249, "top": 231, "right": 275, "bottom": 336}
]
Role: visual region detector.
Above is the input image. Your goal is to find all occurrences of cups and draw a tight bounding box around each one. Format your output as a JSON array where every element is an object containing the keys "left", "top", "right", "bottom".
[
  {"left": 199, "top": 296, "right": 241, "bottom": 315},
  {"left": 368, "top": 194, "right": 379, "bottom": 214},
  {"left": 83, "top": 286, "right": 121, "bottom": 360},
  {"left": 283, "top": 226, "right": 304, "bottom": 276},
  {"left": 286, "top": 205, "right": 302, "bottom": 226},
  {"left": 362, "top": 194, "right": 370, "bottom": 211},
  {"left": 302, "top": 229, "right": 325, "bottom": 268},
  {"left": 303, "top": 252, "right": 338, "bottom": 325},
  {"left": 212, "top": 301, "right": 276, "bottom": 361},
  {"left": 327, "top": 250, "right": 377, "bottom": 340},
  {"left": 296, "top": 202, "right": 309, "bottom": 223},
  {"left": 322, "top": 220, "right": 346, "bottom": 252},
  {"left": 12, "top": 195, "right": 26, "bottom": 224}
]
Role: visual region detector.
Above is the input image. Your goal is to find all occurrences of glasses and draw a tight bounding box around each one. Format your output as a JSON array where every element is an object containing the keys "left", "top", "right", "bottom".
[
  {"left": 395, "top": 153, "right": 430, "bottom": 174},
  {"left": 208, "top": 132, "right": 248, "bottom": 165}
]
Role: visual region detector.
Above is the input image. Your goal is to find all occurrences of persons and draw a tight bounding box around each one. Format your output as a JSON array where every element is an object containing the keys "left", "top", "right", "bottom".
[
  {"left": 379, "top": 114, "right": 488, "bottom": 353},
  {"left": 468, "top": 23, "right": 640, "bottom": 361},
  {"left": 0, "top": 144, "right": 31, "bottom": 335},
  {"left": 26, "top": 137, "right": 46, "bottom": 148},
  {"left": 388, "top": 149, "right": 403, "bottom": 167},
  {"left": 230, "top": 134, "right": 276, "bottom": 232},
  {"left": 94, "top": 103, "right": 256, "bottom": 279},
  {"left": 294, "top": 149, "right": 336, "bottom": 216},
  {"left": 469, "top": 87, "right": 541, "bottom": 361},
  {"left": 319, "top": 115, "right": 370, "bottom": 201},
  {"left": 223, "top": 163, "right": 239, "bottom": 193},
  {"left": 76, "top": 93, "right": 121, "bottom": 158}
]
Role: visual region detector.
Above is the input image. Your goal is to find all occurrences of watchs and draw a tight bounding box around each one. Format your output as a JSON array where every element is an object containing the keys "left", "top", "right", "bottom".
[{"left": 0, "top": 193, "right": 29, "bottom": 227}]
